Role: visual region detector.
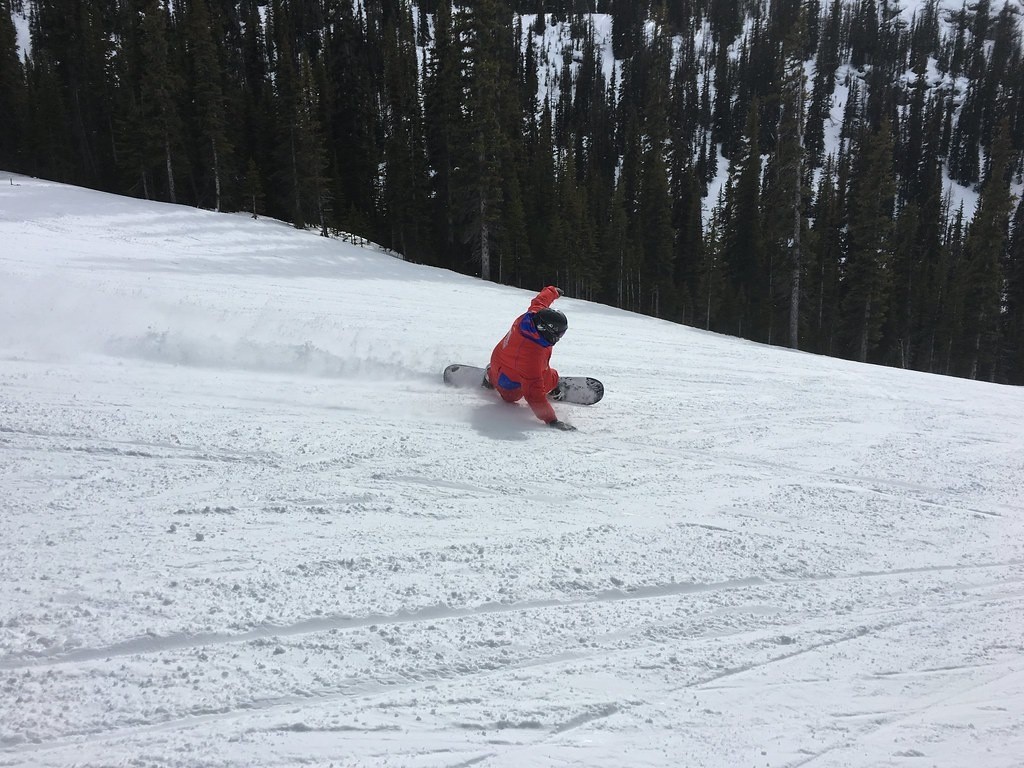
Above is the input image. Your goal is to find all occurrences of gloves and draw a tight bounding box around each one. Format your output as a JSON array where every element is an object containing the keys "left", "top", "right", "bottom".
[
  {"left": 550, "top": 420, "right": 576, "bottom": 432},
  {"left": 555, "top": 287, "right": 564, "bottom": 297}
]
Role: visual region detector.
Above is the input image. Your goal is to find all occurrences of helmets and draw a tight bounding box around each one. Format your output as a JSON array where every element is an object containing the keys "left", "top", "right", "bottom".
[{"left": 534, "top": 307, "right": 568, "bottom": 344}]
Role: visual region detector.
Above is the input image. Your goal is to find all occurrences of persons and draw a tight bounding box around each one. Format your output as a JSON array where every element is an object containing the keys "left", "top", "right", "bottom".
[{"left": 482, "top": 285, "right": 577, "bottom": 431}]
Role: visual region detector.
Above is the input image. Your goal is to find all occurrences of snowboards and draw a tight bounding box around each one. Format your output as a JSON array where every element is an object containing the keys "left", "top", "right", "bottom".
[{"left": 444, "top": 363, "right": 605, "bottom": 406}]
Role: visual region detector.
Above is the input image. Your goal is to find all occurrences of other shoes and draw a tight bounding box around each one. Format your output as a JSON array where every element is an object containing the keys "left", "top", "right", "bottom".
[{"left": 549, "top": 386, "right": 563, "bottom": 401}]
